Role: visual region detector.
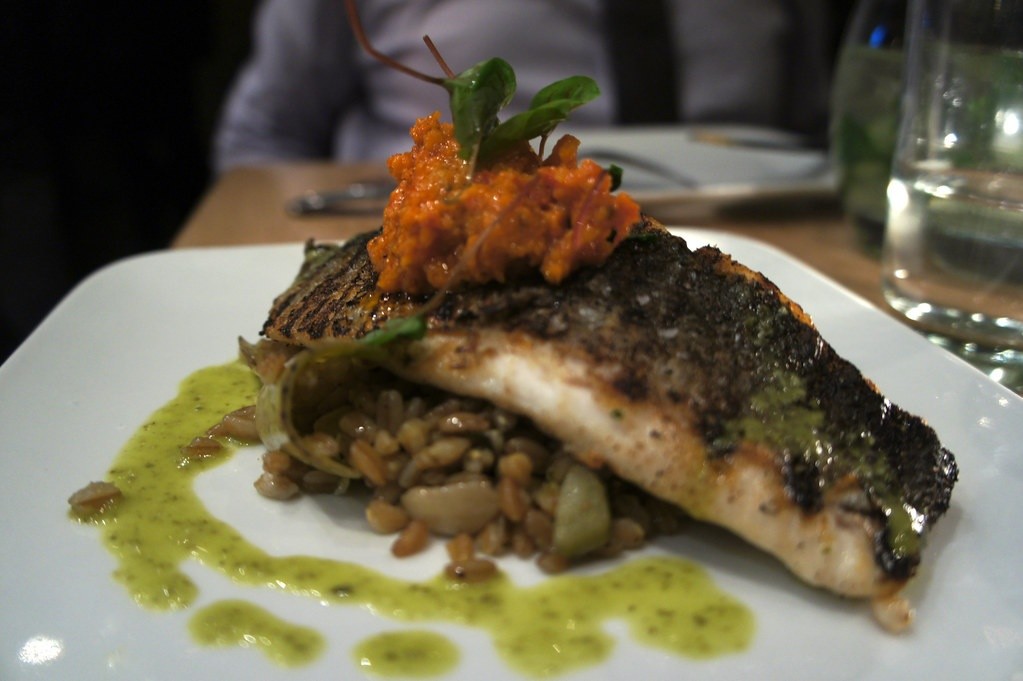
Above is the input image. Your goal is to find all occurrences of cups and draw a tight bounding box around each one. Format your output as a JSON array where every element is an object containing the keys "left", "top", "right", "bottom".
[{"left": 832, "top": 2, "right": 1023, "bottom": 394}]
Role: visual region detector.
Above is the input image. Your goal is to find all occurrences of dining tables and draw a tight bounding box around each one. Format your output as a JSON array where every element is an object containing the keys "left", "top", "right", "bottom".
[{"left": 173, "top": 163, "right": 1023, "bottom": 318}]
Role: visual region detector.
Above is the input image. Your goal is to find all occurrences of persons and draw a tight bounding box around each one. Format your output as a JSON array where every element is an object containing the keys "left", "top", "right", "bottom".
[{"left": 214, "top": 0, "right": 790, "bottom": 171}]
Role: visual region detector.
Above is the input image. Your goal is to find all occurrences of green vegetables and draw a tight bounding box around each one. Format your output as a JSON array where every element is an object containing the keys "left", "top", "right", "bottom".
[{"left": 347, "top": 0, "right": 623, "bottom": 349}]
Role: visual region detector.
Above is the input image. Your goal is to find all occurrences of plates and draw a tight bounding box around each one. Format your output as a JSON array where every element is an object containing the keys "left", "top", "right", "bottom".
[
  {"left": 528, "top": 125, "right": 830, "bottom": 224},
  {"left": 0, "top": 225, "right": 1022, "bottom": 680}
]
